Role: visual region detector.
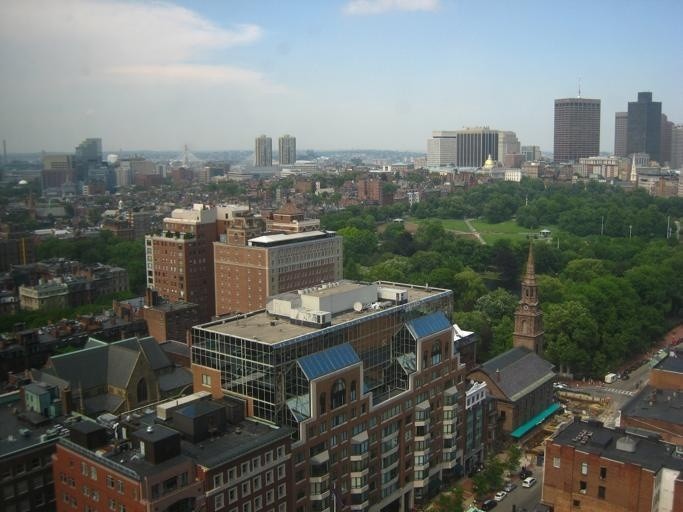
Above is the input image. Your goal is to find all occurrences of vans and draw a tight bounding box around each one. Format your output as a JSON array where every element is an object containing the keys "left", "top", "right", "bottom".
[{"left": 520, "top": 476, "right": 536, "bottom": 488}]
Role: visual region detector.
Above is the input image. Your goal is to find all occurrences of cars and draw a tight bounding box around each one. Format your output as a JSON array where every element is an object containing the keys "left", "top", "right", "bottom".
[
  {"left": 502, "top": 483, "right": 517, "bottom": 493},
  {"left": 552, "top": 382, "right": 568, "bottom": 389},
  {"left": 0, "top": 295, "right": 18, "bottom": 304},
  {"left": 519, "top": 470, "right": 532, "bottom": 480},
  {"left": 480, "top": 499, "right": 498, "bottom": 511},
  {"left": 493, "top": 490, "right": 507, "bottom": 501}
]
